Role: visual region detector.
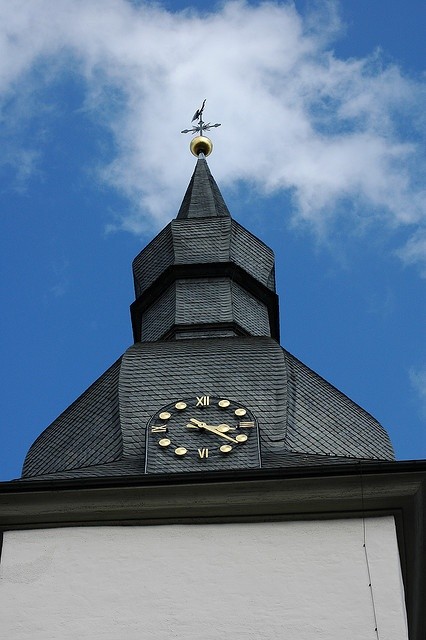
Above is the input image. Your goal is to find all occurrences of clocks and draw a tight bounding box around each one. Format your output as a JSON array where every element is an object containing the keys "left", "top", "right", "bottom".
[{"left": 144, "top": 395, "right": 263, "bottom": 473}]
